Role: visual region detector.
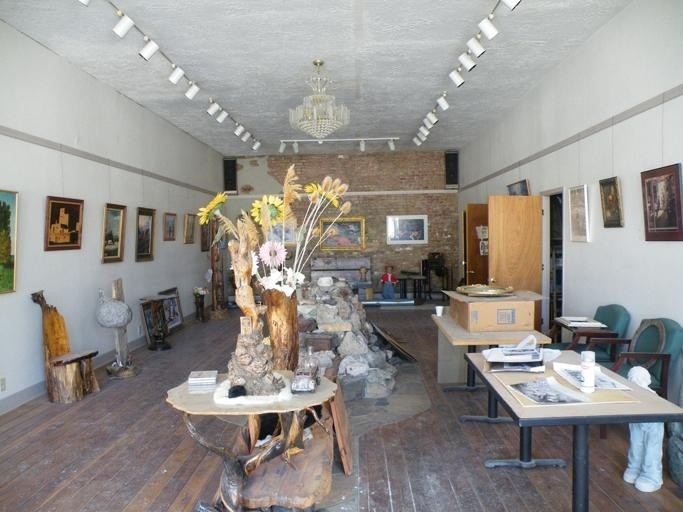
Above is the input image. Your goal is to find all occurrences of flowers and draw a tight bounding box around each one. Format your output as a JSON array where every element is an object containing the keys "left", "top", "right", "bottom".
[
  {"left": 191, "top": 285, "right": 210, "bottom": 296},
  {"left": 194, "top": 163, "right": 356, "bottom": 293}
]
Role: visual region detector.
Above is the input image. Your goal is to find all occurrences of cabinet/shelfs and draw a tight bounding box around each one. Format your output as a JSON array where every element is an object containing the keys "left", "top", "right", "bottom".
[{"left": 420, "top": 263, "right": 450, "bottom": 302}]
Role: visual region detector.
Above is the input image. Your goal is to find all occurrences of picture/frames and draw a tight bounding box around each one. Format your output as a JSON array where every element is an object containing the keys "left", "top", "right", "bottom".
[
  {"left": 570, "top": 185, "right": 588, "bottom": 242},
  {"left": 602, "top": 176, "right": 625, "bottom": 228},
  {"left": 507, "top": 179, "right": 531, "bottom": 196},
  {"left": 44, "top": 195, "right": 216, "bottom": 268},
  {"left": 139, "top": 303, "right": 153, "bottom": 346},
  {"left": 0, "top": 187, "right": 19, "bottom": 295},
  {"left": 639, "top": 163, "right": 682, "bottom": 242},
  {"left": 318, "top": 216, "right": 365, "bottom": 252},
  {"left": 385, "top": 214, "right": 429, "bottom": 245},
  {"left": 157, "top": 285, "right": 184, "bottom": 335}
]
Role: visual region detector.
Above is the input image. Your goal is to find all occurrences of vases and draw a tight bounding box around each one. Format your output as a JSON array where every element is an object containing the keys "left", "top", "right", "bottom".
[
  {"left": 195, "top": 295, "right": 204, "bottom": 322},
  {"left": 258, "top": 287, "right": 299, "bottom": 367}
]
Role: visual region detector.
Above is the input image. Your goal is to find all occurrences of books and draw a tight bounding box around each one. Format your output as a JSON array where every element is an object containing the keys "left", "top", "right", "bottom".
[
  {"left": 555, "top": 316, "right": 602, "bottom": 328},
  {"left": 185, "top": 370, "right": 219, "bottom": 385}
]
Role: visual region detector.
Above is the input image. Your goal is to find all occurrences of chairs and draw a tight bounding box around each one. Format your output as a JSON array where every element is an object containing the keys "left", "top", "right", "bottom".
[
  {"left": 550, "top": 302, "right": 630, "bottom": 352},
  {"left": 597, "top": 318, "right": 683, "bottom": 441}
]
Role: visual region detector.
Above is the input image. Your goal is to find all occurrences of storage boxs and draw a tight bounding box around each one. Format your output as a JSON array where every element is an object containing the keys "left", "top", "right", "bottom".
[{"left": 439, "top": 288, "right": 543, "bottom": 332}]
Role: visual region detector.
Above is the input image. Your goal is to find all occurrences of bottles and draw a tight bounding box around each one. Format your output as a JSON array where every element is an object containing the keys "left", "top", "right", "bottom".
[{"left": 302, "top": 344, "right": 320, "bottom": 384}]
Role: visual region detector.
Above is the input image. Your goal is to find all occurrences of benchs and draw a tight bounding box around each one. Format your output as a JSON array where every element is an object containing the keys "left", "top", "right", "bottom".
[{"left": 47, "top": 349, "right": 100, "bottom": 408}]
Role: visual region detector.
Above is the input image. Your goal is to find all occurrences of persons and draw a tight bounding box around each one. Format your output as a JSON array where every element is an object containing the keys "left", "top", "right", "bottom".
[
  {"left": 379, "top": 265, "right": 397, "bottom": 299},
  {"left": 621, "top": 366, "right": 665, "bottom": 493}
]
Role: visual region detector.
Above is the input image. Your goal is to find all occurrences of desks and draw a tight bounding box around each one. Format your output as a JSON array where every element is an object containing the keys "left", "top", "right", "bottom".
[
  {"left": 349, "top": 282, "right": 372, "bottom": 302},
  {"left": 464, "top": 351, "right": 683, "bottom": 512},
  {"left": 140, "top": 293, "right": 176, "bottom": 350},
  {"left": 394, "top": 274, "right": 424, "bottom": 302},
  {"left": 166, "top": 368, "right": 337, "bottom": 511},
  {"left": 431, "top": 312, "right": 547, "bottom": 423}
]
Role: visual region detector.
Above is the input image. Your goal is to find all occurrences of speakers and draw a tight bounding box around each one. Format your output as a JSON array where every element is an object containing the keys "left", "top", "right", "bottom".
[
  {"left": 444, "top": 150, "right": 460, "bottom": 189},
  {"left": 223, "top": 158, "right": 237, "bottom": 194}
]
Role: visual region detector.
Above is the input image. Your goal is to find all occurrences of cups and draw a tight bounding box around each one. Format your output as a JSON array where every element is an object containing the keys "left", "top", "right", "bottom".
[
  {"left": 435, "top": 305, "right": 443, "bottom": 318},
  {"left": 580, "top": 352, "right": 595, "bottom": 393}
]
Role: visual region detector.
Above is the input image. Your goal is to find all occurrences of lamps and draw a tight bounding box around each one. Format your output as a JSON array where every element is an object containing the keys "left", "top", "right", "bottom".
[
  {"left": 138, "top": 35, "right": 160, "bottom": 62},
  {"left": 111, "top": 9, "right": 136, "bottom": 40},
  {"left": 448, "top": 67, "right": 465, "bottom": 88},
  {"left": 412, "top": 136, "right": 422, "bottom": 147},
  {"left": 359, "top": 140, "right": 366, "bottom": 152},
  {"left": 458, "top": 50, "right": 478, "bottom": 73},
  {"left": 423, "top": 117, "right": 434, "bottom": 130},
  {"left": 387, "top": 139, "right": 395, "bottom": 151},
  {"left": 184, "top": 79, "right": 201, "bottom": 101},
  {"left": 167, "top": 63, "right": 185, "bottom": 86},
  {"left": 215, "top": 110, "right": 229, "bottom": 124},
  {"left": 287, "top": 58, "right": 351, "bottom": 146},
  {"left": 239, "top": 130, "right": 252, "bottom": 143},
  {"left": 74, "top": 0, "right": 92, "bottom": 8},
  {"left": 206, "top": 96, "right": 222, "bottom": 117},
  {"left": 426, "top": 108, "right": 440, "bottom": 128},
  {"left": 476, "top": 13, "right": 503, "bottom": 42},
  {"left": 250, "top": 139, "right": 262, "bottom": 151},
  {"left": 418, "top": 125, "right": 431, "bottom": 137},
  {"left": 436, "top": 90, "right": 451, "bottom": 112},
  {"left": 498, "top": 0, "right": 523, "bottom": 12},
  {"left": 233, "top": 122, "right": 245, "bottom": 137},
  {"left": 465, "top": 34, "right": 490, "bottom": 59},
  {"left": 416, "top": 132, "right": 427, "bottom": 142},
  {"left": 292, "top": 141, "right": 299, "bottom": 154},
  {"left": 278, "top": 141, "right": 287, "bottom": 154}
]
